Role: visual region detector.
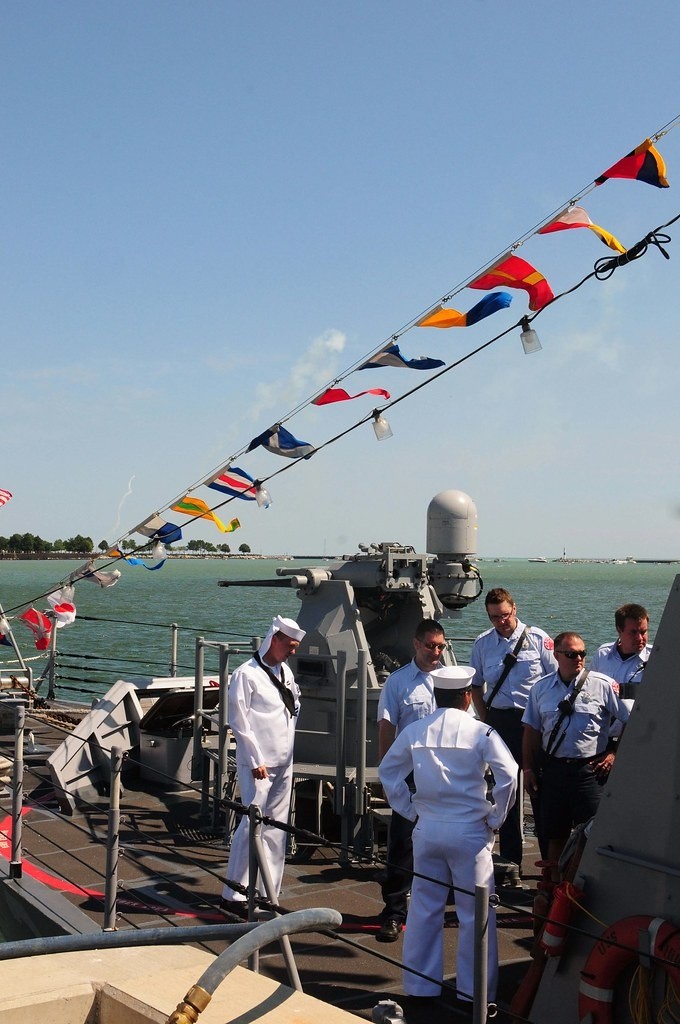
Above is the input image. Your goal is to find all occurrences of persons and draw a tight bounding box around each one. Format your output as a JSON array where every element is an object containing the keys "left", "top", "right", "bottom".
[
  {"left": 377, "top": 665, "right": 521, "bottom": 1019},
  {"left": 380, "top": 622, "right": 491, "bottom": 943},
  {"left": 471, "top": 588, "right": 566, "bottom": 892},
  {"left": 588, "top": 605, "right": 654, "bottom": 717},
  {"left": 220, "top": 613, "right": 306, "bottom": 918},
  {"left": 524, "top": 634, "right": 635, "bottom": 862}
]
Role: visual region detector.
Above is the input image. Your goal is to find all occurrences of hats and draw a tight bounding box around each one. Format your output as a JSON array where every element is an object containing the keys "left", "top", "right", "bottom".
[
  {"left": 429, "top": 665, "right": 476, "bottom": 689},
  {"left": 271, "top": 615, "right": 306, "bottom": 642}
]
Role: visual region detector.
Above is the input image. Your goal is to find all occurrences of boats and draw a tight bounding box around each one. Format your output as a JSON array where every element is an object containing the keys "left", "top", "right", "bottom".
[
  {"left": 258, "top": 555, "right": 266, "bottom": 559},
  {"left": 528, "top": 557, "right": 547, "bottom": 562},
  {"left": 610, "top": 556, "right": 637, "bottom": 564},
  {"left": 277, "top": 558, "right": 287, "bottom": 561}
]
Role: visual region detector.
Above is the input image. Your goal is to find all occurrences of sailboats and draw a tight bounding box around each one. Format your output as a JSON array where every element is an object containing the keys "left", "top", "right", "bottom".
[{"left": 322, "top": 537, "right": 329, "bottom": 562}]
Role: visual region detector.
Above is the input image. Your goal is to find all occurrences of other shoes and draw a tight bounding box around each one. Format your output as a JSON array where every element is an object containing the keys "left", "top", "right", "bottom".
[{"left": 219, "top": 897, "right": 250, "bottom": 918}]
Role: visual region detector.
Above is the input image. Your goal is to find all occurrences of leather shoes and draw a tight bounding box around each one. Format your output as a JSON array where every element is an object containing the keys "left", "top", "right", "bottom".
[{"left": 375, "top": 919, "right": 403, "bottom": 942}]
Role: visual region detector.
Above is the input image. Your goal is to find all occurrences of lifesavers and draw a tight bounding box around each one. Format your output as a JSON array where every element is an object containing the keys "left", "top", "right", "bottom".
[{"left": 579, "top": 915, "right": 680, "bottom": 1024}]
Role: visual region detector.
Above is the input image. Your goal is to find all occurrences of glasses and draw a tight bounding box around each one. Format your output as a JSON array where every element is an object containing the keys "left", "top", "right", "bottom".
[
  {"left": 422, "top": 641, "right": 447, "bottom": 651},
  {"left": 557, "top": 650, "right": 586, "bottom": 659},
  {"left": 488, "top": 606, "right": 513, "bottom": 623}
]
